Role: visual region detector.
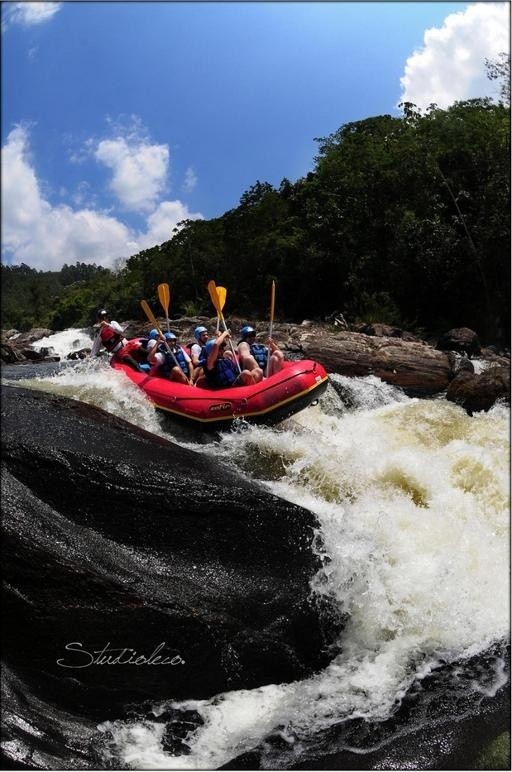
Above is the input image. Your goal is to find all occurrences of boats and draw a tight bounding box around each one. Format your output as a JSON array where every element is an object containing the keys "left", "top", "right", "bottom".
[{"left": 109, "top": 336, "right": 328, "bottom": 432}]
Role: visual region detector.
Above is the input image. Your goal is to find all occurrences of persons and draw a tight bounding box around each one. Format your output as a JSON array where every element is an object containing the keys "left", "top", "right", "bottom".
[
  {"left": 89, "top": 309, "right": 149, "bottom": 373},
  {"left": 147, "top": 323, "right": 285, "bottom": 390}
]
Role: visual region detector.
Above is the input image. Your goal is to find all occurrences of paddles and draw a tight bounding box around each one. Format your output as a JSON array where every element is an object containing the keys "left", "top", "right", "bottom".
[
  {"left": 208, "top": 281, "right": 242, "bottom": 375},
  {"left": 267, "top": 280, "right": 277, "bottom": 377},
  {"left": 157, "top": 283, "right": 170, "bottom": 333},
  {"left": 140, "top": 299, "right": 190, "bottom": 384}
]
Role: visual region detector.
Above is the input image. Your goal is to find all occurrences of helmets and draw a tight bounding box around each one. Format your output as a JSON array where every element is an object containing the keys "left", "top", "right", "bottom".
[
  {"left": 194, "top": 326, "right": 208, "bottom": 341},
  {"left": 97, "top": 309, "right": 108, "bottom": 318},
  {"left": 150, "top": 328, "right": 159, "bottom": 339},
  {"left": 240, "top": 326, "right": 256, "bottom": 337},
  {"left": 163, "top": 332, "right": 177, "bottom": 340}
]
